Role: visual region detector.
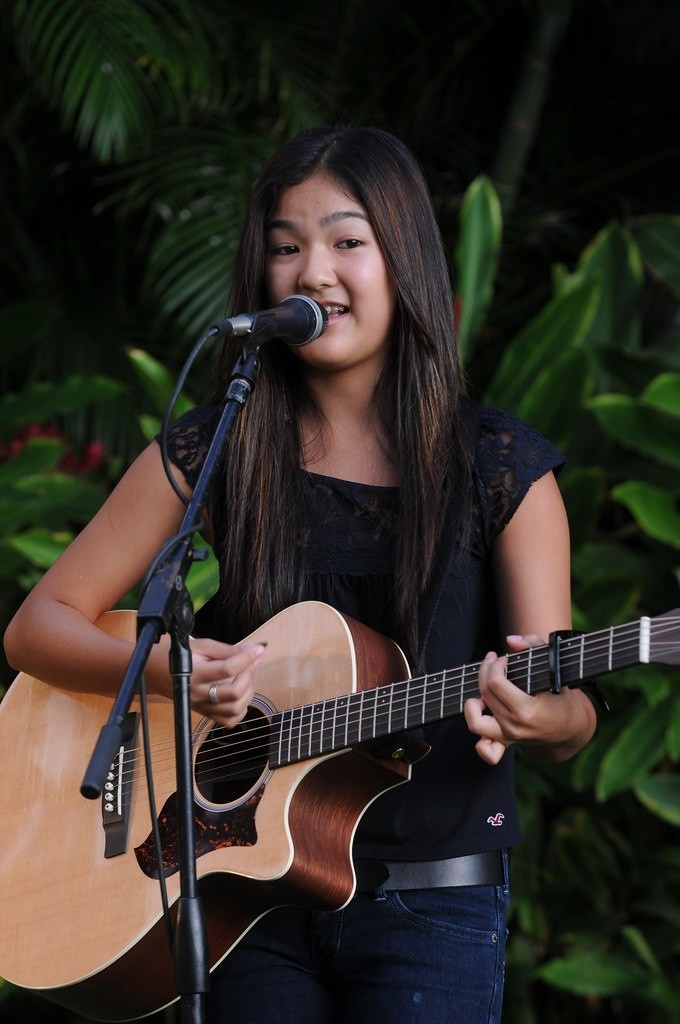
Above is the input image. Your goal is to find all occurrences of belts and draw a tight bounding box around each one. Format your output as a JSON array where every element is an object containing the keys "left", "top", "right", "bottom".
[{"left": 351, "top": 849, "right": 511, "bottom": 894}]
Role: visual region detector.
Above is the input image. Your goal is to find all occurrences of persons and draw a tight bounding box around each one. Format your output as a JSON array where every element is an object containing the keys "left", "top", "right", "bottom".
[{"left": 3, "top": 118, "right": 597, "bottom": 1024}]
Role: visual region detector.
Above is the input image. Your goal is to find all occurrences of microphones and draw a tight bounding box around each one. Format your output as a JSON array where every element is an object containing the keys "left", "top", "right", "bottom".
[{"left": 211, "top": 294, "right": 330, "bottom": 347}]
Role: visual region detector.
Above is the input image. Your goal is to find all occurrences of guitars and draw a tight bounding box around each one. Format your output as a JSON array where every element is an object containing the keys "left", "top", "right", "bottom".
[{"left": 0, "top": 598, "right": 680, "bottom": 1024}]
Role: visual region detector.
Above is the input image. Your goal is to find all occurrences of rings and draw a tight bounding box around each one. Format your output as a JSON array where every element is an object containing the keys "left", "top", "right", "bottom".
[{"left": 209, "top": 683, "right": 220, "bottom": 704}]
